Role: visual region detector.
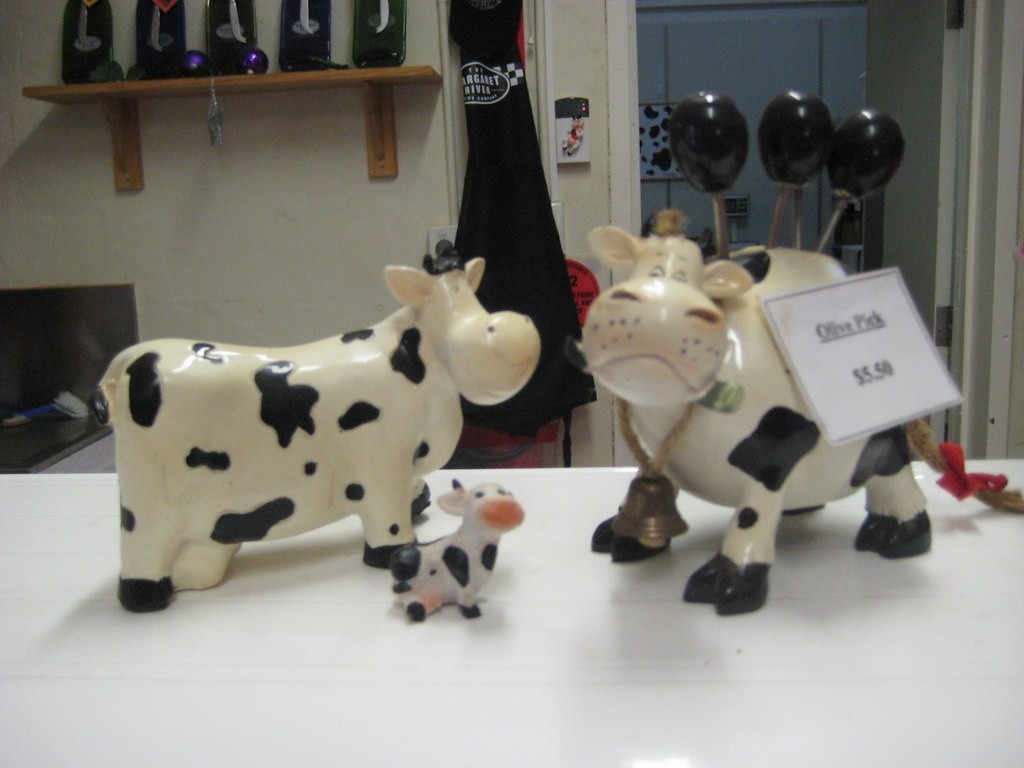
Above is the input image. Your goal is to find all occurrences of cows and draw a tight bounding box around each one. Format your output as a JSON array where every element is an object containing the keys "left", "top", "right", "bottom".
[
  {"left": 100, "top": 239, "right": 541, "bottom": 613},
  {"left": 390, "top": 478, "right": 525, "bottom": 622},
  {"left": 584, "top": 226, "right": 932, "bottom": 617}
]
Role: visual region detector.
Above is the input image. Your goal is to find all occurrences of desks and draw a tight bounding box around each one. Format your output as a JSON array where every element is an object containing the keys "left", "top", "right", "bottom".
[{"left": 0, "top": 462, "right": 1024, "bottom": 768}]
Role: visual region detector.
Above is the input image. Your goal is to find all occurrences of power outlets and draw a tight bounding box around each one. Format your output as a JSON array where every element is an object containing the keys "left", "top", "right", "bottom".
[{"left": 429, "top": 225, "right": 457, "bottom": 258}]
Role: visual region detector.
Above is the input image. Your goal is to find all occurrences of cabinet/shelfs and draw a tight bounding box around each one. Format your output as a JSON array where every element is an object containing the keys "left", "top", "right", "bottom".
[{"left": 635, "top": 0, "right": 868, "bottom": 253}]
